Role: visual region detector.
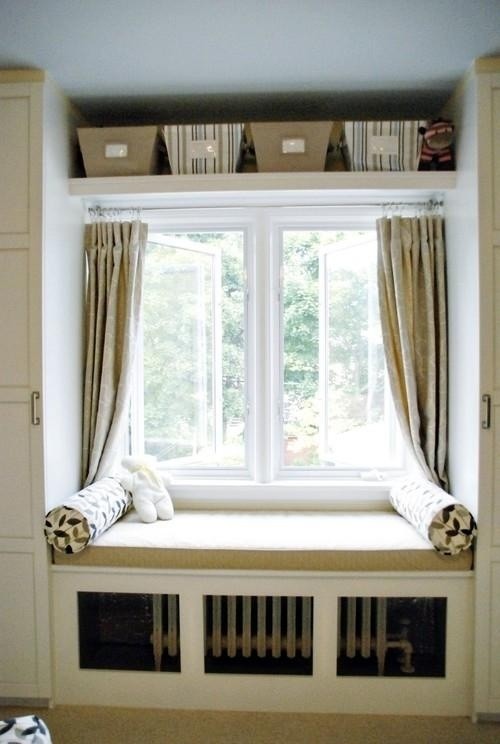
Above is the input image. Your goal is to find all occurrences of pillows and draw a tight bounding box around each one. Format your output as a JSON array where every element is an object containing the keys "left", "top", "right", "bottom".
[
  {"left": 387, "top": 475, "right": 481, "bottom": 559},
  {"left": 37, "top": 475, "right": 137, "bottom": 557}
]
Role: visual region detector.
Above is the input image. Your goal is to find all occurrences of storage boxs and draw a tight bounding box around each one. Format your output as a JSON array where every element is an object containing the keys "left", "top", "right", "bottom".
[
  {"left": 342, "top": 120, "right": 428, "bottom": 172},
  {"left": 247, "top": 121, "right": 336, "bottom": 174},
  {"left": 74, "top": 125, "right": 160, "bottom": 175},
  {"left": 159, "top": 122, "right": 246, "bottom": 175}
]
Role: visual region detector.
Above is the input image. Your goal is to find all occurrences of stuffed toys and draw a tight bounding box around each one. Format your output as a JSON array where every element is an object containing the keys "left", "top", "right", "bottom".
[
  {"left": 418, "top": 117, "right": 456, "bottom": 172},
  {"left": 116, "top": 453, "right": 175, "bottom": 523}
]
